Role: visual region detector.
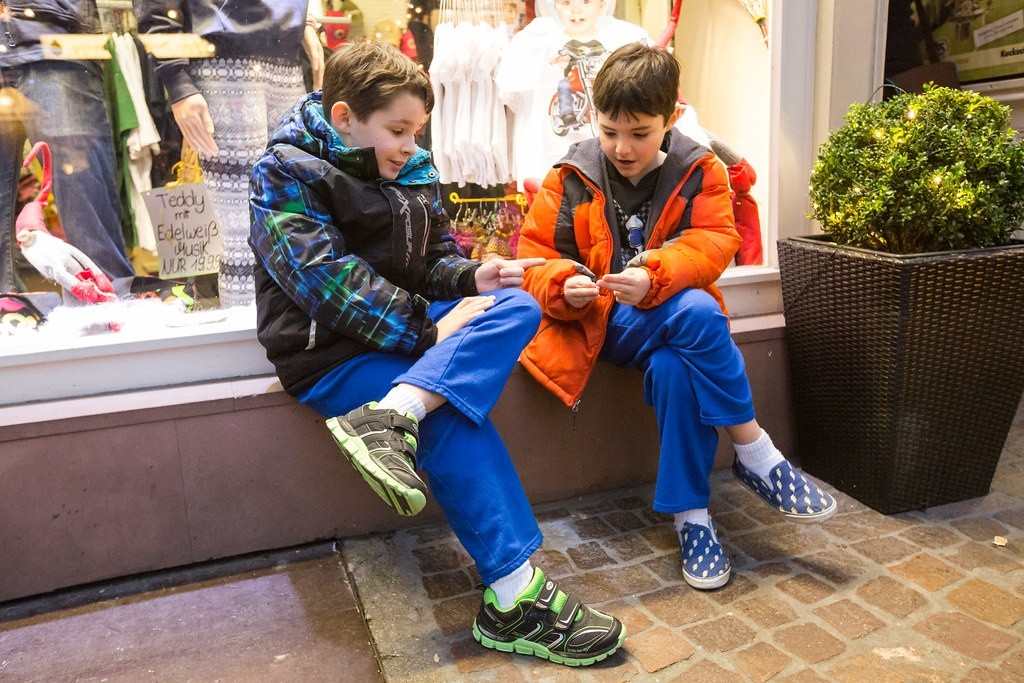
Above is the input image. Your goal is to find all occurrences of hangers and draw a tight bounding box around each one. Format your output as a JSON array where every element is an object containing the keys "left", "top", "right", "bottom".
[
  {"left": 451, "top": 200, "right": 521, "bottom": 236},
  {"left": 438, "top": 0, "right": 504, "bottom": 29}
]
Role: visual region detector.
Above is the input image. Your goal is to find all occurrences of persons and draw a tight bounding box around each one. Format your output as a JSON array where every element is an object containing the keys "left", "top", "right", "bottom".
[
  {"left": 516, "top": 42, "right": 837, "bottom": 588},
  {"left": 553, "top": 0, "right": 606, "bottom": 40},
  {"left": 247, "top": 44, "right": 626, "bottom": 667},
  {"left": 1, "top": 0, "right": 134, "bottom": 326},
  {"left": 132, "top": 0, "right": 325, "bottom": 310}
]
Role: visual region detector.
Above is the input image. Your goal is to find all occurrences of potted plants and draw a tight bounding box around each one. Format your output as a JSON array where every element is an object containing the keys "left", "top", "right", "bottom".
[{"left": 775, "top": 81, "right": 1024, "bottom": 516}]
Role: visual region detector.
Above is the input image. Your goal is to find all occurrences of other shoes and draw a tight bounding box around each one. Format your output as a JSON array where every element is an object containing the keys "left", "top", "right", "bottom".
[
  {"left": 674, "top": 511, "right": 731, "bottom": 589},
  {"left": 732, "top": 449, "right": 837, "bottom": 523}
]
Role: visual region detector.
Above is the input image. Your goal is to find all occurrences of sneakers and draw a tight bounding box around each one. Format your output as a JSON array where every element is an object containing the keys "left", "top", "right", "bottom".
[
  {"left": 326, "top": 401, "right": 429, "bottom": 516},
  {"left": 472, "top": 567, "right": 626, "bottom": 666}
]
